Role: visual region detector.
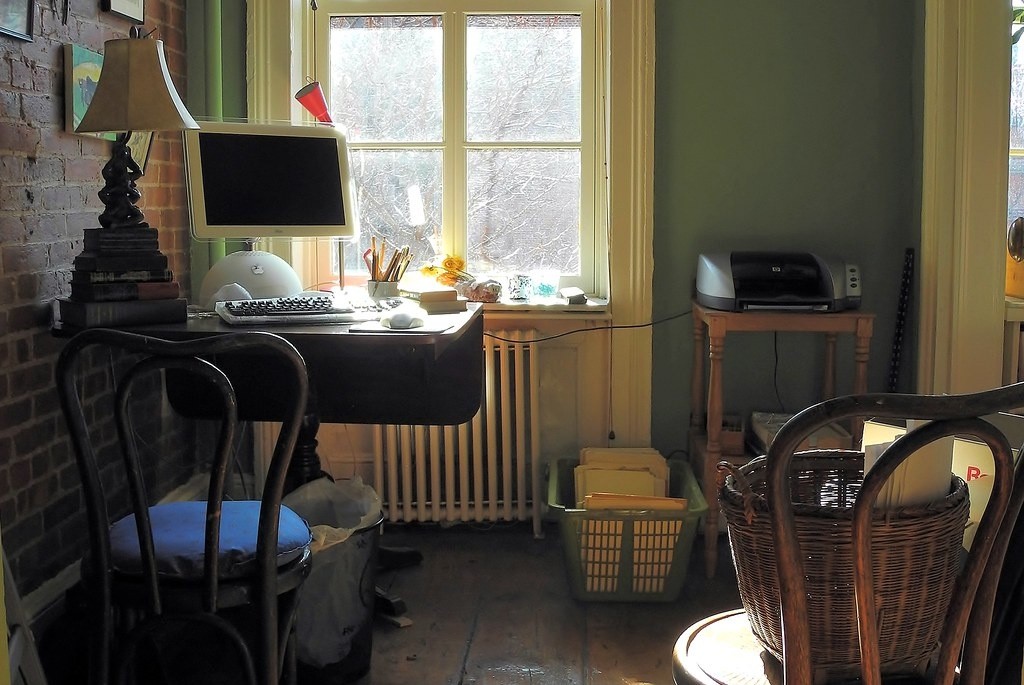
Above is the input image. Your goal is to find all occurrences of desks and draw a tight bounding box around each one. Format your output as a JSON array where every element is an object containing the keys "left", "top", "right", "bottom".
[{"left": 55, "top": 302, "right": 484, "bottom": 614}]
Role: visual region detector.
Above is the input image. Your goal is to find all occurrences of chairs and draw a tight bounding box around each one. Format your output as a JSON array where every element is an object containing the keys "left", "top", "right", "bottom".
[{"left": 54, "top": 329, "right": 313, "bottom": 685}]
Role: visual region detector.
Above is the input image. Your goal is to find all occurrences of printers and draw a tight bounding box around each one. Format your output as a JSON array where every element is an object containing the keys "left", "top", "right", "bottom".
[{"left": 696, "top": 254, "right": 863, "bottom": 314}]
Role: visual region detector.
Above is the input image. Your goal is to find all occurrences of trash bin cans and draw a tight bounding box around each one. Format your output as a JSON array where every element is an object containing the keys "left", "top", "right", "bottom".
[{"left": 256, "top": 497, "right": 384, "bottom": 685}]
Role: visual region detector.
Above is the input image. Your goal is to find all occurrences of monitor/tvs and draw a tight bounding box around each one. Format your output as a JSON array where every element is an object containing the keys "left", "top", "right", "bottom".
[{"left": 184, "top": 121, "right": 354, "bottom": 239}]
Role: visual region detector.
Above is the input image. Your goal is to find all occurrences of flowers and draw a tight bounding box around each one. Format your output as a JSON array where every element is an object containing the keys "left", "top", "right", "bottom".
[{"left": 421, "top": 256, "right": 502, "bottom": 303}]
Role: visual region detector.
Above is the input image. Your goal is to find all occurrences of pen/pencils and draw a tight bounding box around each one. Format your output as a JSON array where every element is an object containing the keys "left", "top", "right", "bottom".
[{"left": 371, "top": 235, "right": 414, "bottom": 282}]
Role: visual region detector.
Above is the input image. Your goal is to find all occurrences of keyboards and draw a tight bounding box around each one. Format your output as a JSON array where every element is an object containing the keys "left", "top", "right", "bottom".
[{"left": 215, "top": 293, "right": 381, "bottom": 324}]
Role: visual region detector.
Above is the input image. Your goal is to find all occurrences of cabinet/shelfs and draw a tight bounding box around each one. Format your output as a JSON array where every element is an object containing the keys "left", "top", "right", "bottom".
[{"left": 688, "top": 299, "right": 875, "bottom": 581}]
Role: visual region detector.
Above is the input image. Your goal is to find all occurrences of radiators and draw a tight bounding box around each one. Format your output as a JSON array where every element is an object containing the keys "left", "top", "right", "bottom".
[{"left": 371, "top": 330, "right": 549, "bottom": 538}]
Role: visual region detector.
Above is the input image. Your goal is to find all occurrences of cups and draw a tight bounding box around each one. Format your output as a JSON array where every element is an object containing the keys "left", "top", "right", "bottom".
[
  {"left": 367, "top": 280, "right": 398, "bottom": 306},
  {"left": 530, "top": 269, "right": 561, "bottom": 305},
  {"left": 506, "top": 273, "right": 531, "bottom": 302}
]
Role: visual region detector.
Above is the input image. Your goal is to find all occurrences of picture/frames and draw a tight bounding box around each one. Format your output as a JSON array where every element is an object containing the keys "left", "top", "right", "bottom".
[
  {"left": 102, "top": 0, "right": 145, "bottom": 25},
  {"left": 0, "top": 0, "right": 35, "bottom": 43}
]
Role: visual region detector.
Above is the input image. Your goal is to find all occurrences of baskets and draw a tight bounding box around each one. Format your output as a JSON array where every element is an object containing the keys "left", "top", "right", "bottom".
[{"left": 714, "top": 450, "right": 971, "bottom": 673}]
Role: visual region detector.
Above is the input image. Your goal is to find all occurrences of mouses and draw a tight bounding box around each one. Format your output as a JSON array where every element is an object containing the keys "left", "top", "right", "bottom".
[{"left": 379, "top": 303, "right": 428, "bottom": 329}]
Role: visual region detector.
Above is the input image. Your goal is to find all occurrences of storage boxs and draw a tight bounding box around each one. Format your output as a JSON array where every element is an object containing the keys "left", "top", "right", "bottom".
[
  {"left": 721, "top": 413, "right": 746, "bottom": 456},
  {"left": 549, "top": 456, "right": 707, "bottom": 606}
]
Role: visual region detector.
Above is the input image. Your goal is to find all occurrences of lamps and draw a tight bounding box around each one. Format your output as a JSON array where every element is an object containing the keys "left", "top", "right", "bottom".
[
  {"left": 73, "top": 27, "right": 200, "bottom": 250},
  {"left": 295, "top": 77, "right": 345, "bottom": 292}
]
